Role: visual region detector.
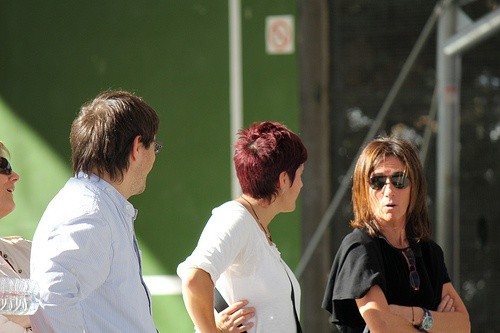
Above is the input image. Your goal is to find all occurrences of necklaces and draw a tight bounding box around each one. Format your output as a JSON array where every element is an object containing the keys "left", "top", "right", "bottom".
[{"left": 241, "top": 195, "right": 273, "bottom": 241}]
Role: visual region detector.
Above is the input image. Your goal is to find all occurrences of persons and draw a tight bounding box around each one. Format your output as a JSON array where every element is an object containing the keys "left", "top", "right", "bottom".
[
  {"left": 320, "top": 136, "right": 471, "bottom": 333},
  {"left": 177, "top": 120, "right": 308, "bottom": 333},
  {"left": 0, "top": 143, "right": 32, "bottom": 333},
  {"left": 30, "top": 87, "right": 160, "bottom": 332}
]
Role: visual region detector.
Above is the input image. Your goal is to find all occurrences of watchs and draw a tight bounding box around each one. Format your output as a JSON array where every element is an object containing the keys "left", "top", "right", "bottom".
[{"left": 414, "top": 309, "right": 433, "bottom": 331}]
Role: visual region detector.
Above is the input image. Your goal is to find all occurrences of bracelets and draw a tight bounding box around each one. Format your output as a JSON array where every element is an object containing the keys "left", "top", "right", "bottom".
[{"left": 411, "top": 306, "right": 414, "bottom": 326}]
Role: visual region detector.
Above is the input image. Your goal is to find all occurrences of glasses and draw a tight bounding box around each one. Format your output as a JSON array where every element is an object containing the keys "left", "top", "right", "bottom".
[
  {"left": 0, "top": 157, "right": 12, "bottom": 175},
  {"left": 402, "top": 247, "right": 420, "bottom": 291},
  {"left": 140, "top": 132, "right": 163, "bottom": 155},
  {"left": 368, "top": 175, "right": 411, "bottom": 190}
]
